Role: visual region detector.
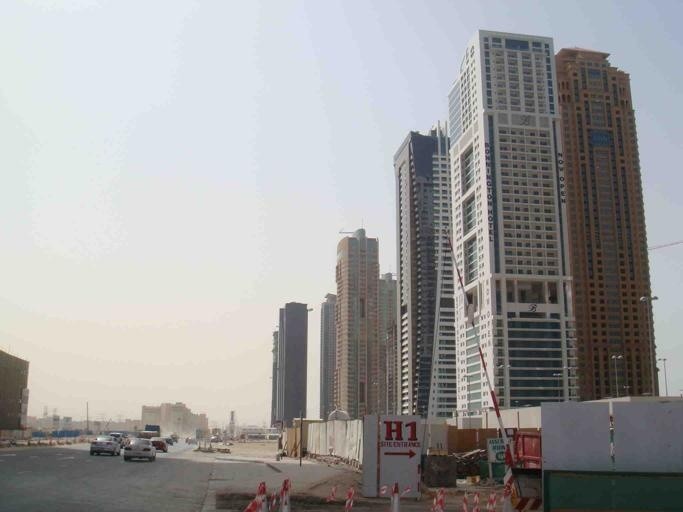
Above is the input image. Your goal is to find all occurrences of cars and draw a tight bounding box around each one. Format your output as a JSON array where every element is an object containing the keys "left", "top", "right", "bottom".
[{"left": 90, "top": 433, "right": 168, "bottom": 462}]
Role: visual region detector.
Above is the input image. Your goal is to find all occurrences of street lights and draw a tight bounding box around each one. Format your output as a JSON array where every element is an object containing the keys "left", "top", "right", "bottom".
[
  {"left": 611, "top": 355, "right": 623, "bottom": 396},
  {"left": 658, "top": 357, "right": 668, "bottom": 397},
  {"left": 553, "top": 373, "right": 563, "bottom": 402},
  {"left": 640, "top": 296, "right": 658, "bottom": 396}
]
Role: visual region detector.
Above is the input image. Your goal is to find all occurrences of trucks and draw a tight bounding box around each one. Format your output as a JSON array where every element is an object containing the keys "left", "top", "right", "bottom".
[{"left": 138, "top": 425, "right": 160, "bottom": 438}]
